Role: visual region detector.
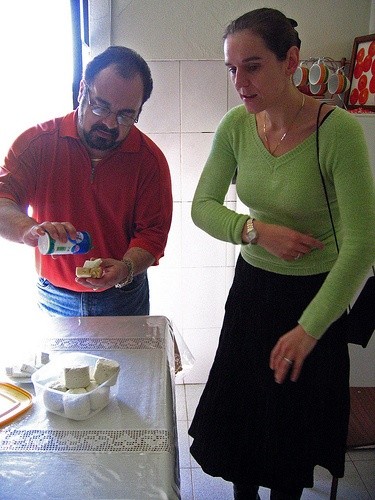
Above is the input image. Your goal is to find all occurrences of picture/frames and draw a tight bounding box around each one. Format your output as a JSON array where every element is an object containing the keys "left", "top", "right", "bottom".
[{"left": 342, "top": 33, "right": 375, "bottom": 113}]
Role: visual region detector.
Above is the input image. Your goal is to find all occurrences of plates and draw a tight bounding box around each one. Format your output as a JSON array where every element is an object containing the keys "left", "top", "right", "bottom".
[{"left": 7, "top": 354, "right": 54, "bottom": 383}]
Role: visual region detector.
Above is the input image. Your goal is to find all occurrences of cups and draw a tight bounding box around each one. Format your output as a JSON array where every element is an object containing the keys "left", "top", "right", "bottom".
[
  {"left": 308, "top": 59, "right": 332, "bottom": 85},
  {"left": 291, "top": 61, "right": 310, "bottom": 87},
  {"left": 328, "top": 68, "right": 351, "bottom": 95},
  {"left": 309, "top": 82, "right": 328, "bottom": 95}
]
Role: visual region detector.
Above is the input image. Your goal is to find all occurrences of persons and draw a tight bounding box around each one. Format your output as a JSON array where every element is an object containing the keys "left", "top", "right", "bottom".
[
  {"left": 0, "top": 46, "right": 173, "bottom": 316},
  {"left": 188, "top": 8, "right": 375, "bottom": 500}
]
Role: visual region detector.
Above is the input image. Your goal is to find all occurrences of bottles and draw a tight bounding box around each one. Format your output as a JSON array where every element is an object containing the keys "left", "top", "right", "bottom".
[{"left": 38, "top": 231, "right": 93, "bottom": 255}]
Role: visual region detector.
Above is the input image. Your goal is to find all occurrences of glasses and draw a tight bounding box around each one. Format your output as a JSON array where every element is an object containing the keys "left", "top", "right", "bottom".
[{"left": 87, "top": 88, "right": 142, "bottom": 127}]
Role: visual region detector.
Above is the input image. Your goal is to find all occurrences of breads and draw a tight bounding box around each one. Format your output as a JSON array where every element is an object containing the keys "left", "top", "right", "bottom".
[
  {"left": 75, "top": 266, "right": 103, "bottom": 278},
  {"left": 42, "top": 358, "right": 120, "bottom": 419}
]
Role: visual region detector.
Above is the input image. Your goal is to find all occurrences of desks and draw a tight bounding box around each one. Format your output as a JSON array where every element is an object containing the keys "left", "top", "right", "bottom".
[{"left": 1, "top": 314, "right": 197, "bottom": 500}]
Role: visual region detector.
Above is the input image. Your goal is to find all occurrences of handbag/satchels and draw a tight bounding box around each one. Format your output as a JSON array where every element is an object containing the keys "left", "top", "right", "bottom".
[{"left": 335, "top": 275, "right": 375, "bottom": 348}]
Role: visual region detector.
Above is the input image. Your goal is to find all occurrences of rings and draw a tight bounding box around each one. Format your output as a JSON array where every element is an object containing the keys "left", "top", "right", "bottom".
[
  {"left": 278, "top": 352, "right": 285, "bottom": 359},
  {"left": 284, "top": 357, "right": 294, "bottom": 365},
  {"left": 93, "top": 287, "right": 100, "bottom": 291},
  {"left": 292, "top": 252, "right": 300, "bottom": 260}
]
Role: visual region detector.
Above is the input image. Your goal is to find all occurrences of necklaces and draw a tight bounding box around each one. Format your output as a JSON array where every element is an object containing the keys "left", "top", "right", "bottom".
[
  {"left": 90, "top": 157, "right": 104, "bottom": 162},
  {"left": 263, "top": 92, "right": 305, "bottom": 156}
]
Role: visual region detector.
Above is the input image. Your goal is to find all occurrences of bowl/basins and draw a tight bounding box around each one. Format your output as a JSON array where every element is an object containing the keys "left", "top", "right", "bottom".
[{"left": 31, "top": 351, "right": 120, "bottom": 420}]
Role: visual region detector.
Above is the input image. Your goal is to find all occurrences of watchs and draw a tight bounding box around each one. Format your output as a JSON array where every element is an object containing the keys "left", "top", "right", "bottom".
[
  {"left": 245, "top": 218, "right": 258, "bottom": 245},
  {"left": 115, "top": 257, "right": 133, "bottom": 288}
]
